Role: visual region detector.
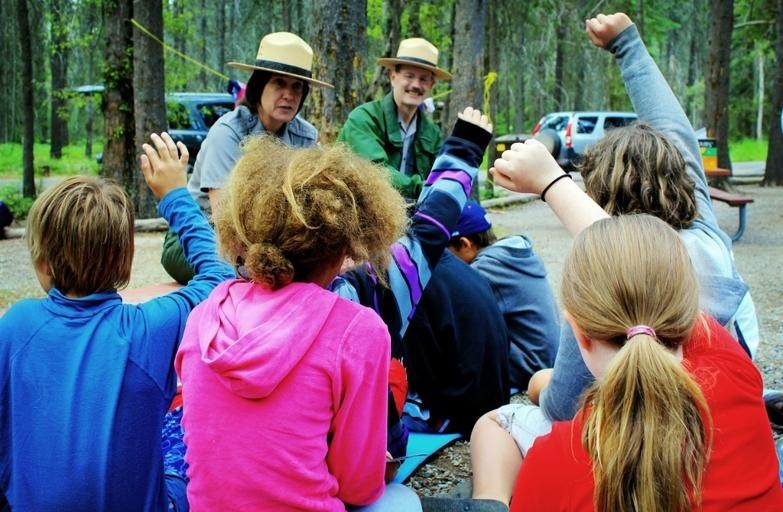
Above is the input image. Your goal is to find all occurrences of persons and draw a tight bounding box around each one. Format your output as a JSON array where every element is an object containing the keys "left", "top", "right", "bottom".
[
  {"left": 490, "top": 138, "right": 783, "bottom": 512},
  {"left": 335, "top": 36, "right": 454, "bottom": 209},
  {"left": 161, "top": 32, "right": 335, "bottom": 284},
  {"left": 419, "top": 12, "right": 783, "bottom": 512},
  {"left": 173, "top": 133, "right": 424, "bottom": 512},
  {"left": 2, "top": 131, "right": 241, "bottom": 511},
  {"left": 400, "top": 203, "right": 511, "bottom": 435},
  {"left": 446, "top": 199, "right": 561, "bottom": 392},
  {"left": 325, "top": 105, "right": 494, "bottom": 484}
]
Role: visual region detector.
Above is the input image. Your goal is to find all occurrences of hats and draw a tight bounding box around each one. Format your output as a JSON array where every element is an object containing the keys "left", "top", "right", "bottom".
[
  {"left": 450, "top": 200, "right": 492, "bottom": 238},
  {"left": 377, "top": 38, "right": 454, "bottom": 80},
  {"left": 232, "top": 32, "right": 335, "bottom": 89}
]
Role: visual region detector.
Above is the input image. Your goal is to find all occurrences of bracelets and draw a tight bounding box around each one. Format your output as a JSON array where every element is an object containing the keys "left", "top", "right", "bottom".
[{"left": 540, "top": 173, "right": 572, "bottom": 202}]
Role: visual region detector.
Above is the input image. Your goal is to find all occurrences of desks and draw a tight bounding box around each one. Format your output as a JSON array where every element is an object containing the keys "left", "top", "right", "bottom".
[{"left": 705, "top": 168, "right": 730, "bottom": 177}]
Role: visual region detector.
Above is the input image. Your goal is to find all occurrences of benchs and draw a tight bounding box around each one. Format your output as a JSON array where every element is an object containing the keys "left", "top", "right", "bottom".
[{"left": 709, "top": 185, "right": 755, "bottom": 242}]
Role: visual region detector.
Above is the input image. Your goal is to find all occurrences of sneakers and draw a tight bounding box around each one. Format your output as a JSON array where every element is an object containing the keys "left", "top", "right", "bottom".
[{"left": 763, "top": 390, "right": 782, "bottom": 435}]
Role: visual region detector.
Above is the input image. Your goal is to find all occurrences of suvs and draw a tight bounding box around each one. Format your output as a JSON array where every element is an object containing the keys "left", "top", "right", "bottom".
[
  {"left": 529, "top": 112, "right": 640, "bottom": 172},
  {"left": 77, "top": 85, "right": 235, "bottom": 173}
]
